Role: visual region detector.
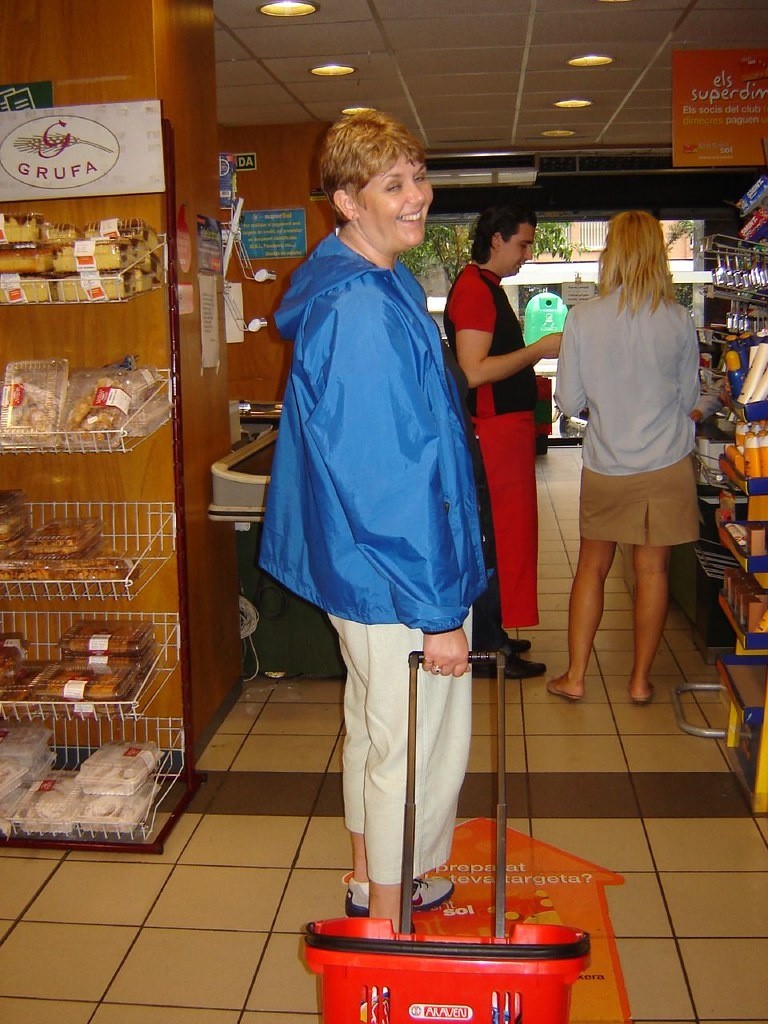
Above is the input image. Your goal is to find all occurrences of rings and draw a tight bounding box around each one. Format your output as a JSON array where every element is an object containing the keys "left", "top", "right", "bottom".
[
  {"left": 425, "top": 657, "right": 431, "bottom": 663},
  {"left": 433, "top": 666, "right": 441, "bottom": 673}
]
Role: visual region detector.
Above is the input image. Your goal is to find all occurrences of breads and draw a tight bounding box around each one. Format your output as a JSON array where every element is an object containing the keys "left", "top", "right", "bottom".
[
  {"left": 0, "top": 212, "right": 161, "bottom": 303},
  {"left": 0, "top": 488, "right": 139, "bottom": 586},
  {"left": 0, "top": 618, "right": 156, "bottom": 719}
]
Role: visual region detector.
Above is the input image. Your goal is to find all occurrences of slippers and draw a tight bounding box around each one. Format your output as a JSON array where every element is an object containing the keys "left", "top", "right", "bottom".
[
  {"left": 628, "top": 681, "right": 654, "bottom": 705},
  {"left": 547, "top": 679, "right": 584, "bottom": 704}
]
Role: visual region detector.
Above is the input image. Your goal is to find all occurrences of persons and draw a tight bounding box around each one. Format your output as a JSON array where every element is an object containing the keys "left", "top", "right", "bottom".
[
  {"left": 257, "top": 107, "right": 489, "bottom": 917},
  {"left": 545, "top": 211, "right": 702, "bottom": 703},
  {"left": 444, "top": 197, "right": 564, "bottom": 678}
]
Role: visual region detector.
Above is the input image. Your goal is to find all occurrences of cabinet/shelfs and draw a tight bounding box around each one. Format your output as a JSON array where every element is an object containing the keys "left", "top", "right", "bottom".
[
  {"left": 0, "top": 101, "right": 207, "bottom": 851},
  {"left": 688, "top": 233, "right": 768, "bottom": 665}
]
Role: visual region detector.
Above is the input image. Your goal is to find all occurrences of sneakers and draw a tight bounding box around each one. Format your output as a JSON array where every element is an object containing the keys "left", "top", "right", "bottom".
[{"left": 345, "top": 877, "right": 454, "bottom": 918}]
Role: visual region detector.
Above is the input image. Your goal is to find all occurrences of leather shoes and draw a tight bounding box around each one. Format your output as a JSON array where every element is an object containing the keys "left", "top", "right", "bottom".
[
  {"left": 472, "top": 655, "right": 547, "bottom": 678},
  {"left": 496, "top": 639, "right": 531, "bottom": 653}
]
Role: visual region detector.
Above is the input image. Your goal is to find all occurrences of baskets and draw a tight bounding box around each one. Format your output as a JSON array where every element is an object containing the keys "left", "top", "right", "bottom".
[{"left": 305, "top": 916, "right": 591, "bottom": 1024}]
